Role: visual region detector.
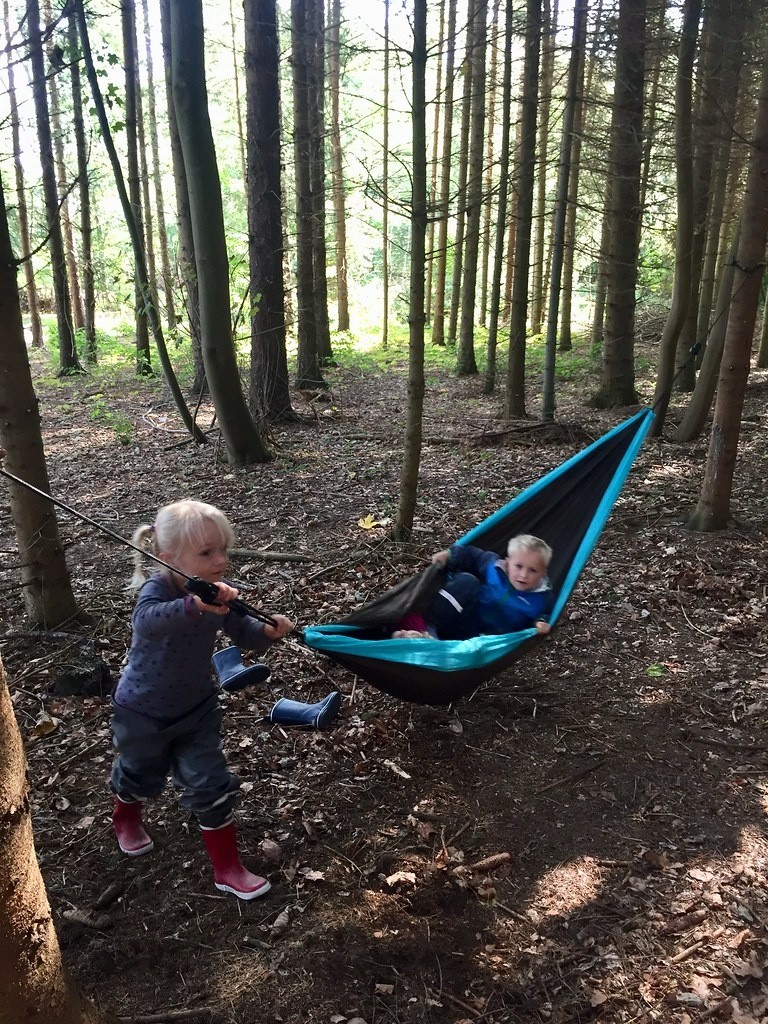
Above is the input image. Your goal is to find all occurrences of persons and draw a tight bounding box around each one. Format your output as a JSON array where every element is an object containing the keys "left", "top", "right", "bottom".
[
  {"left": 107, "top": 499, "right": 294, "bottom": 899},
  {"left": 392, "top": 534, "right": 554, "bottom": 640}
]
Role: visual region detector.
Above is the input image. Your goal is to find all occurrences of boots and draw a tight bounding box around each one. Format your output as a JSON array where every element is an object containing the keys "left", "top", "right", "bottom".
[
  {"left": 211, "top": 645, "right": 271, "bottom": 692},
  {"left": 199, "top": 815, "right": 272, "bottom": 901},
  {"left": 111, "top": 793, "right": 154, "bottom": 857},
  {"left": 269, "top": 690, "right": 342, "bottom": 730}
]
